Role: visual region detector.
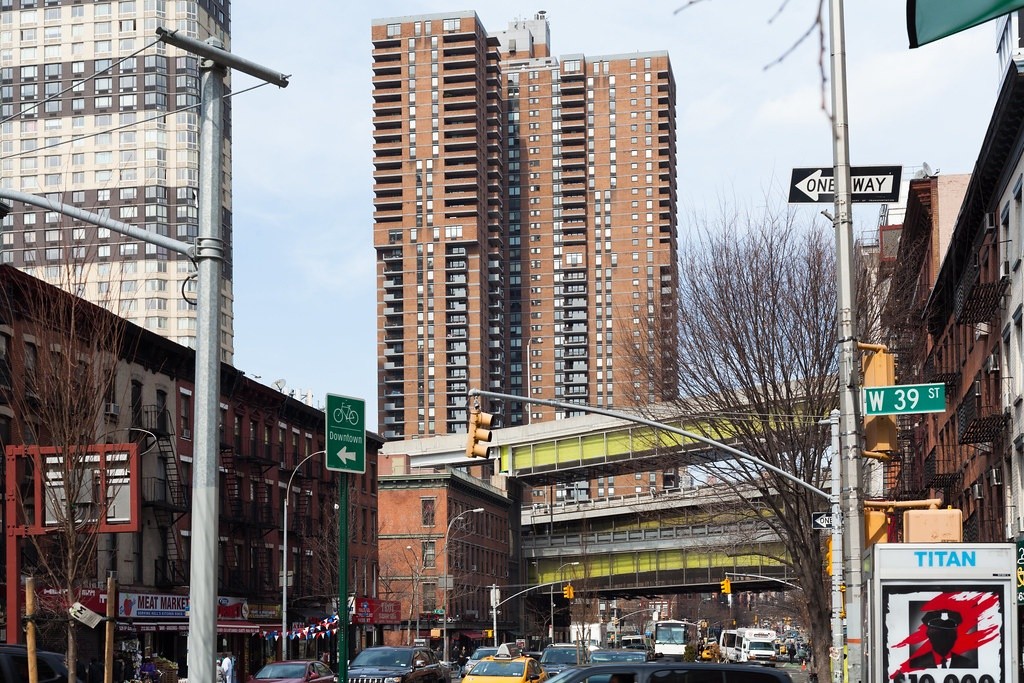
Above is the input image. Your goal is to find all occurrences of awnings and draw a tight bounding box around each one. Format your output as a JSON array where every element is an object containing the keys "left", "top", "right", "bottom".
[
  {"left": 414, "top": 630, "right": 440, "bottom": 640},
  {"left": 132, "top": 622, "right": 189, "bottom": 632},
  {"left": 906, "top": 0, "right": 1024, "bottom": 49},
  {"left": 216, "top": 620, "right": 260, "bottom": 633},
  {"left": 460, "top": 631, "right": 482, "bottom": 641}
]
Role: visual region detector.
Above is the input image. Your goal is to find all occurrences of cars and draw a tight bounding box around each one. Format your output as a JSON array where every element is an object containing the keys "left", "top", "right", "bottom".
[
  {"left": 702, "top": 638, "right": 717, "bottom": 659},
  {"left": 331, "top": 645, "right": 451, "bottom": 683},
  {"left": 460, "top": 643, "right": 657, "bottom": 683},
  {"left": 247, "top": 660, "right": 338, "bottom": 683},
  {"left": 775, "top": 630, "right": 811, "bottom": 660}
]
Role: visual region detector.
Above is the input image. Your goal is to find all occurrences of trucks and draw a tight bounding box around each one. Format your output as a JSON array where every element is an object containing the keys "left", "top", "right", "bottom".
[
  {"left": 733, "top": 627, "right": 777, "bottom": 667},
  {"left": 719, "top": 630, "right": 738, "bottom": 660},
  {"left": 621, "top": 634, "right": 646, "bottom": 649},
  {"left": 570, "top": 623, "right": 608, "bottom": 647}
]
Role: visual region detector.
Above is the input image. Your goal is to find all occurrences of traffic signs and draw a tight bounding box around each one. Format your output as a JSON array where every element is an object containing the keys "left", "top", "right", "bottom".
[
  {"left": 812, "top": 512, "right": 844, "bottom": 529},
  {"left": 721, "top": 579, "right": 731, "bottom": 594},
  {"left": 787, "top": 166, "right": 903, "bottom": 203}
]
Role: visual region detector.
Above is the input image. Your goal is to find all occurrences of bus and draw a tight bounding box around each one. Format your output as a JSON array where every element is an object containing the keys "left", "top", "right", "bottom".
[{"left": 651, "top": 620, "right": 697, "bottom": 656}]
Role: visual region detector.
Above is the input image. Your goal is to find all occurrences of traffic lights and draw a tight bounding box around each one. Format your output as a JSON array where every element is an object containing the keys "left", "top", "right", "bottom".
[
  {"left": 614, "top": 618, "right": 618, "bottom": 624},
  {"left": 563, "top": 586, "right": 570, "bottom": 598},
  {"left": 466, "top": 407, "right": 496, "bottom": 459}
]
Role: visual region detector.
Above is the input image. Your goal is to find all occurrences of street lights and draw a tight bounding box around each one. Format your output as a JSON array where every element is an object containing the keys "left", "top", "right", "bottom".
[
  {"left": 407, "top": 546, "right": 416, "bottom": 639},
  {"left": 551, "top": 562, "right": 579, "bottom": 645},
  {"left": 697, "top": 598, "right": 711, "bottom": 621},
  {"left": 443, "top": 507, "right": 484, "bottom": 661}
]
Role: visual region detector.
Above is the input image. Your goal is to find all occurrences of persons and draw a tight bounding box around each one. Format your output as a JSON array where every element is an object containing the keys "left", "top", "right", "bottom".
[
  {"left": 449, "top": 645, "right": 468, "bottom": 678},
  {"left": 787, "top": 645, "right": 796, "bottom": 664},
  {"left": 798, "top": 647, "right": 806, "bottom": 665},
  {"left": 433, "top": 647, "right": 443, "bottom": 660},
  {"left": 76, "top": 656, "right": 104, "bottom": 683},
  {"left": 216, "top": 652, "right": 236, "bottom": 683}
]
